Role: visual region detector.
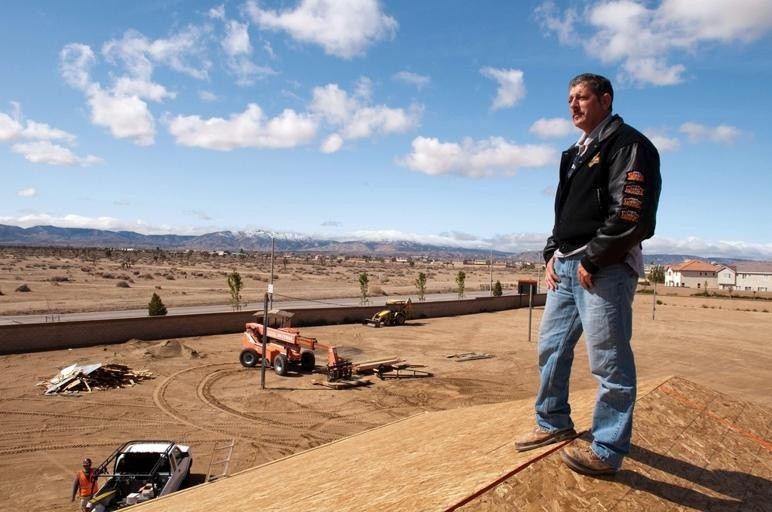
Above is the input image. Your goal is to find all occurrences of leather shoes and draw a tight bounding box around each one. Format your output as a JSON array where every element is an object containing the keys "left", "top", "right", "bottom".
[
  {"left": 557, "top": 438, "right": 619, "bottom": 479},
  {"left": 512, "top": 424, "right": 578, "bottom": 453}
]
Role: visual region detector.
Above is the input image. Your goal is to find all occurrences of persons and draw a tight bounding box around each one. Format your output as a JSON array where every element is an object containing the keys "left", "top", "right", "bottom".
[
  {"left": 514, "top": 70, "right": 662, "bottom": 476},
  {"left": 69, "top": 456, "right": 106, "bottom": 511}
]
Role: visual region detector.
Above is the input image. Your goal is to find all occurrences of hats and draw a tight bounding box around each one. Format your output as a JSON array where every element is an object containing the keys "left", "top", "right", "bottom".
[{"left": 81, "top": 458, "right": 91, "bottom": 467}]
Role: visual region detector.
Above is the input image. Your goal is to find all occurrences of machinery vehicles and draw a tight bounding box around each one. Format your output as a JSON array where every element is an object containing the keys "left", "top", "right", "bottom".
[
  {"left": 362, "top": 297, "right": 412, "bottom": 327},
  {"left": 239, "top": 322, "right": 352, "bottom": 382}
]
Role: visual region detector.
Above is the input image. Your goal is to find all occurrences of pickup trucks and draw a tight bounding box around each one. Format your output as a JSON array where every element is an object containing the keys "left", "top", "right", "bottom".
[{"left": 86, "top": 440, "right": 193, "bottom": 512}]
